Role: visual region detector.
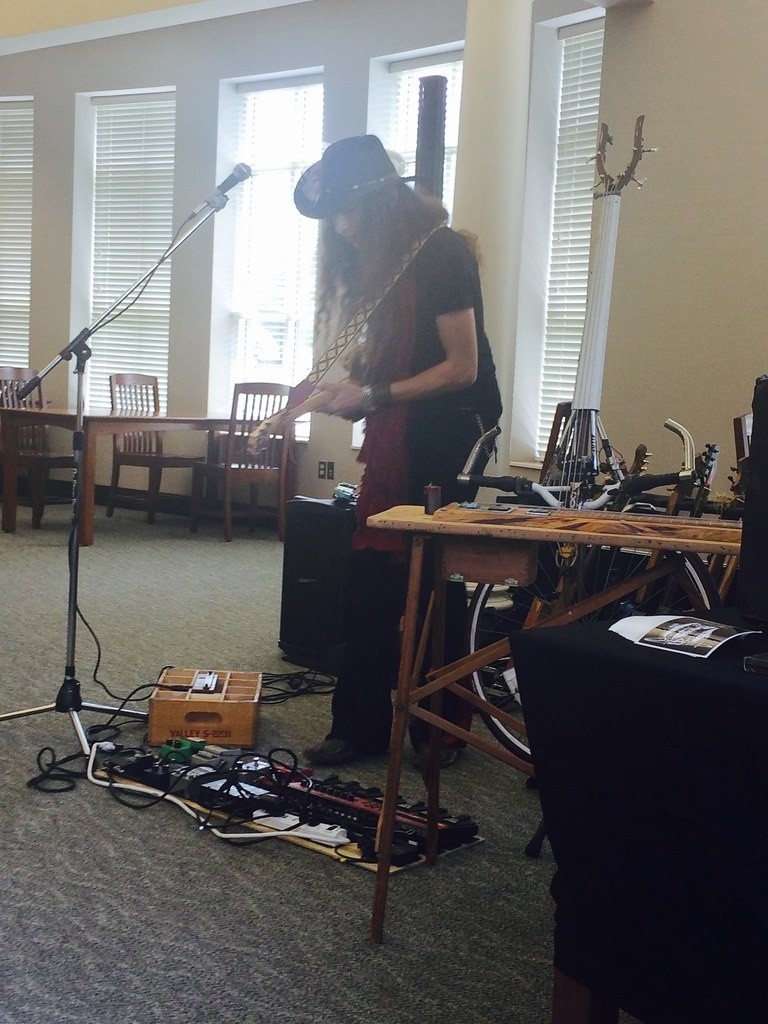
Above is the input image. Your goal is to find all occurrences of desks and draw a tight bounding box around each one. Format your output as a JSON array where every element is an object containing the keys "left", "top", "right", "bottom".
[
  {"left": 1, "top": 406, "right": 295, "bottom": 547},
  {"left": 510, "top": 609, "right": 768, "bottom": 1023},
  {"left": 364, "top": 503, "right": 743, "bottom": 940}
]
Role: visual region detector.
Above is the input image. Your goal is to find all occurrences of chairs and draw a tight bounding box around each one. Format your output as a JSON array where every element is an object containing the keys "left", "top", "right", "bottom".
[
  {"left": 0, "top": 366, "right": 76, "bottom": 531},
  {"left": 104, "top": 373, "right": 206, "bottom": 527},
  {"left": 734, "top": 411, "right": 754, "bottom": 480},
  {"left": 189, "top": 383, "right": 293, "bottom": 539},
  {"left": 541, "top": 401, "right": 593, "bottom": 485}
]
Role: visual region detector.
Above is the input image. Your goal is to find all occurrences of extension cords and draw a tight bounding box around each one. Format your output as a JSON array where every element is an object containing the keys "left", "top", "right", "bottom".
[{"left": 253, "top": 809, "right": 347, "bottom": 846}]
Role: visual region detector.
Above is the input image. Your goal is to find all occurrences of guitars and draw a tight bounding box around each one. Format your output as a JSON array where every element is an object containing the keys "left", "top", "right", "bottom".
[
  {"left": 587, "top": 443, "right": 751, "bottom": 621},
  {"left": 246, "top": 340, "right": 366, "bottom": 456}
]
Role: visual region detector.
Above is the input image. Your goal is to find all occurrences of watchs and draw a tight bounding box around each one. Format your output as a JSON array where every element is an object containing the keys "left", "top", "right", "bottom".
[{"left": 360, "top": 383, "right": 377, "bottom": 418}]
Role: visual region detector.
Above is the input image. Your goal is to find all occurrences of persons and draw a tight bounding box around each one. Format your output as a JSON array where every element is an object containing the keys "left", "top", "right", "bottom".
[{"left": 294, "top": 136, "right": 503, "bottom": 769}]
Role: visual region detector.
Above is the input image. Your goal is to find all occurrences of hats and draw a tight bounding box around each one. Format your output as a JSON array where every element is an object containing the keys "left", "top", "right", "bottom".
[{"left": 294, "top": 134, "right": 422, "bottom": 219}]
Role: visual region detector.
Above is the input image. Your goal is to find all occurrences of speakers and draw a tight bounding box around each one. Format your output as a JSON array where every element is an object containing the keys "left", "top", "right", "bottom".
[{"left": 278, "top": 498, "right": 361, "bottom": 677}]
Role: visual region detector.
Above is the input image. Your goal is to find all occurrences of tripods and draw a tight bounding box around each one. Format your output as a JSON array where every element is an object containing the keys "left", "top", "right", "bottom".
[{"left": 0, "top": 193, "right": 231, "bottom": 771}]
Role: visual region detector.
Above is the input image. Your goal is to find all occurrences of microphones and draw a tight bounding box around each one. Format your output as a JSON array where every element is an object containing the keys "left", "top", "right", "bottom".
[{"left": 187, "top": 163, "right": 251, "bottom": 222}]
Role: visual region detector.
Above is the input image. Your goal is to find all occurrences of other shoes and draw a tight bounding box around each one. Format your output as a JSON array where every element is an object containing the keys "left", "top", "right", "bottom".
[
  {"left": 416, "top": 741, "right": 454, "bottom": 767},
  {"left": 301, "top": 737, "right": 384, "bottom": 766}
]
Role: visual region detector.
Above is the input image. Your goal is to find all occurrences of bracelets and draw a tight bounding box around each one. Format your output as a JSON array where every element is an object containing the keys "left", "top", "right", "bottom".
[{"left": 373, "top": 379, "right": 394, "bottom": 407}]
